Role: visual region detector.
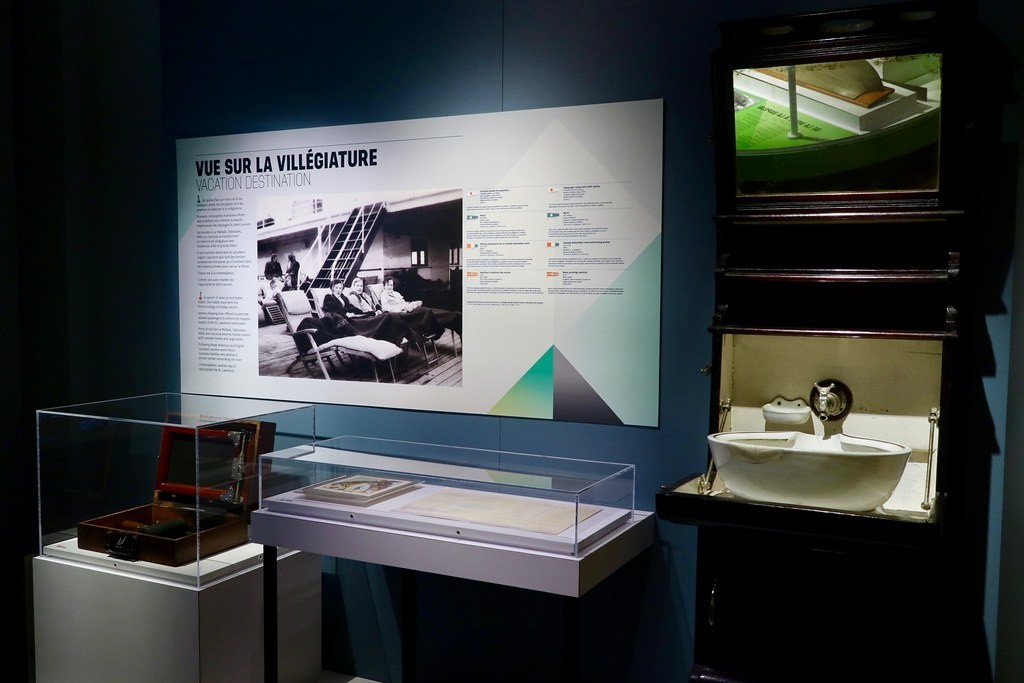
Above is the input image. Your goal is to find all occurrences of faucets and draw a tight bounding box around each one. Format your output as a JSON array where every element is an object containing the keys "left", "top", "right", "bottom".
[{"left": 813, "top": 383, "right": 847, "bottom": 422}]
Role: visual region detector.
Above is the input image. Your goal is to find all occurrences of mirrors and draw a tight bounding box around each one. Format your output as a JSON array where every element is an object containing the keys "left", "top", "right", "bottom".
[{"left": 732, "top": 50, "right": 945, "bottom": 199}]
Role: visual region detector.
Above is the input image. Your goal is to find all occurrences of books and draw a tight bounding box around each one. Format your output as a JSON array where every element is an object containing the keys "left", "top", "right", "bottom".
[{"left": 303, "top": 468, "right": 426, "bottom": 509}]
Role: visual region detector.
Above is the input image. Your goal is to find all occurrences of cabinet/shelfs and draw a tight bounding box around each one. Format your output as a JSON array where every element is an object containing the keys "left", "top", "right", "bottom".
[{"left": 659, "top": 0, "right": 1024, "bottom": 683}]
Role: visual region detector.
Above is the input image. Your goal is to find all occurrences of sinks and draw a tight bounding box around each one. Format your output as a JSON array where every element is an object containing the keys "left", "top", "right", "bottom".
[{"left": 707, "top": 431, "right": 913, "bottom": 512}]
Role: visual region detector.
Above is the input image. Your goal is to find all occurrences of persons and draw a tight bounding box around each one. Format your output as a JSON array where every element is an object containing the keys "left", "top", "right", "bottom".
[
  {"left": 264, "top": 251, "right": 300, "bottom": 290},
  {"left": 324, "top": 275, "right": 462, "bottom": 347}
]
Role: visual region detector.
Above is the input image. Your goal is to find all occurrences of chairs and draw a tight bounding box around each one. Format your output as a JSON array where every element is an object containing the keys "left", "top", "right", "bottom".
[{"left": 274, "top": 283, "right": 462, "bottom": 384}]
